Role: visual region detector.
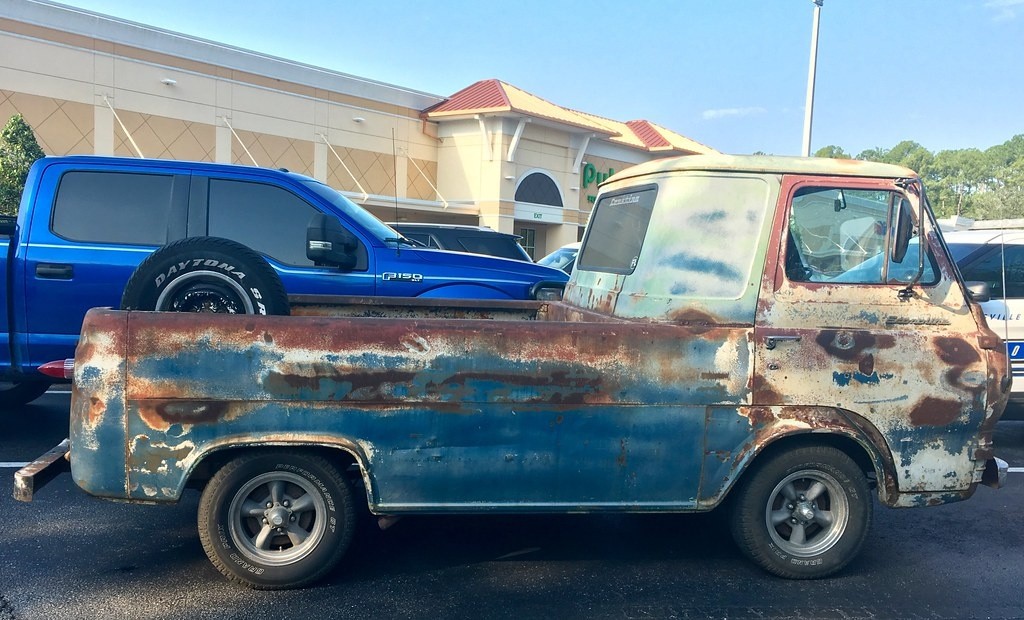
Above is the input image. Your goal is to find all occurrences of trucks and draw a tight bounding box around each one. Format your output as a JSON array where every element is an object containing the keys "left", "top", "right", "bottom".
[{"left": 13, "top": 155, "right": 1013, "bottom": 592}]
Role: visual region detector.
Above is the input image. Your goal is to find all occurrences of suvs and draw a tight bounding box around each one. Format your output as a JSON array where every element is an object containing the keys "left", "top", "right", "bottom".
[
  {"left": 383, "top": 222, "right": 535, "bottom": 263},
  {"left": 822, "top": 216, "right": 1024, "bottom": 423}
]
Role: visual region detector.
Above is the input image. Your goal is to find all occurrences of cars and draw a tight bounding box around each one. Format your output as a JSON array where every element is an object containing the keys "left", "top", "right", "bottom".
[{"left": 535, "top": 242, "right": 581, "bottom": 275}]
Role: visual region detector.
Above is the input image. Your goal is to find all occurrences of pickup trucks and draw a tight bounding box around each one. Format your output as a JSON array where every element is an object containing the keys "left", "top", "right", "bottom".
[{"left": 0, "top": 157, "right": 573, "bottom": 408}]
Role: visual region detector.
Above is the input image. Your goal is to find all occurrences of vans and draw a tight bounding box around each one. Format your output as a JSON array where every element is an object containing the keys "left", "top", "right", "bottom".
[{"left": 793, "top": 191, "right": 901, "bottom": 278}]
